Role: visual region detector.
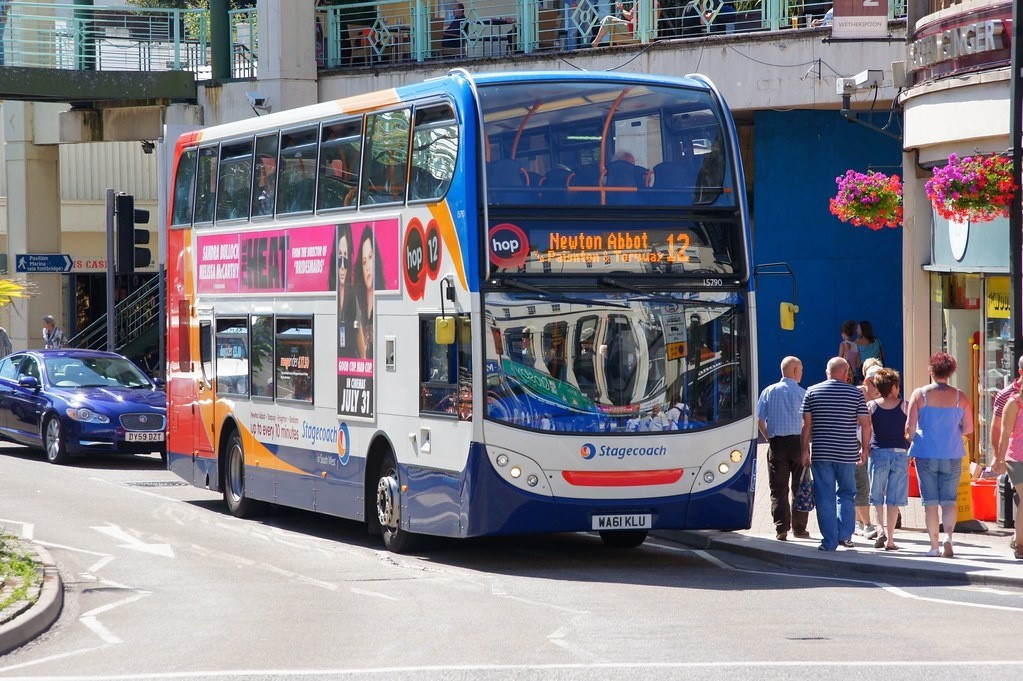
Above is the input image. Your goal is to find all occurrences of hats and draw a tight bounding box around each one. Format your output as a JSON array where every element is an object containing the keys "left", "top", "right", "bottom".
[{"left": 43, "top": 316, "right": 56, "bottom": 325}]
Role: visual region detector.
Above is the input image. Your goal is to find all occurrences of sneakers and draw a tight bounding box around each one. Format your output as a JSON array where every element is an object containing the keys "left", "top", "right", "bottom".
[
  {"left": 864, "top": 526, "right": 877, "bottom": 538},
  {"left": 854, "top": 520, "right": 864, "bottom": 536}
]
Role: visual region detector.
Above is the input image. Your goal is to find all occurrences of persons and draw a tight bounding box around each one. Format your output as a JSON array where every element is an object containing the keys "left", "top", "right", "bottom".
[
  {"left": 681, "top": 0, "right": 714, "bottom": 38},
  {"left": 799, "top": 357, "right": 872, "bottom": 551},
  {"left": 42, "top": 315, "right": 68, "bottom": 350},
  {"left": 859, "top": 369, "right": 910, "bottom": 550},
  {"left": 0, "top": 327, "right": 11, "bottom": 360},
  {"left": 591, "top": 0, "right": 660, "bottom": 48},
  {"left": 353, "top": 225, "right": 385, "bottom": 362},
  {"left": 329, "top": 224, "right": 354, "bottom": 365},
  {"left": 997, "top": 383, "right": 1023, "bottom": 559},
  {"left": 837, "top": 321, "right": 902, "bottom": 541},
  {"left": 990, "top": 356, "right": 1023, "bottom": 548},
  {"left": 757, "top": 356, "right": 809, "bottom": 540},
  {"left": 564, "top": 0, "right": 598, "bottom": 49},
  {"left": 905, "top": 352, "right": 973, "bottom": 559},
  {"left": 441, "top": 3, "right": 469, "bottom": 57},
  {"left": 520, "top": 324, "right": 639, "bottom": 394}
]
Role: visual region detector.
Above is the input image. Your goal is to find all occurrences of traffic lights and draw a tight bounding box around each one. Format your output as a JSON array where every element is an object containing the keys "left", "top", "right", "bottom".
[{"left": 115, "top": 195, "right": 152, "bottom": 275}]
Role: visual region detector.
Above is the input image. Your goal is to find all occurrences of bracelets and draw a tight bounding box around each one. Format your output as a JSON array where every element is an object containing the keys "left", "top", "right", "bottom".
[{"left": 996, "top": 459, "right": 1005, "bottom": 464}]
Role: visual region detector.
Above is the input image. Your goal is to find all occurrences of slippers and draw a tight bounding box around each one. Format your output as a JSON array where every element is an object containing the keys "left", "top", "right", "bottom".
[
  {"left": 581, "top": 43, "right": 594, "bottom": 48},
  {"left": 875, "top": 535, "right": 887, "bottom": 547},
  {"left": 886, "top": 545, "right": 898, "bottom": 550}
]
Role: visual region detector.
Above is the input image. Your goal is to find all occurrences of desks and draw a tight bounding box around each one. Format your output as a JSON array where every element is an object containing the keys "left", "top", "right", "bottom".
[
  {"left": 470, "top": 37, "right": 508, "bottom": 56},
  {"left": 470, "top": 19, "right": 517, "bottom": 57},
  {"left": 374, "top": 26, "right": 414, "bottom": 63}
]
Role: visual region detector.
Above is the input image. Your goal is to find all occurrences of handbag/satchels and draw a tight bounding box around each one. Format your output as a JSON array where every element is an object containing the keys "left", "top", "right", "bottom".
[
  {"left": 634, "top": 425, "right": 638, "bottom": 432},
  {"left": 851, "top": 367, "right": 865, "bottom": 386},
  {"left": 795, "top": 466, "right": 814, "bottom": 512}
]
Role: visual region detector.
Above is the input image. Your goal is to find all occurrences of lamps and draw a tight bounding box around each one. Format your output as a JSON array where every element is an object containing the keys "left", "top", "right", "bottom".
[{"left": 141, "top": 140, "right": 155, "bottom": 154}]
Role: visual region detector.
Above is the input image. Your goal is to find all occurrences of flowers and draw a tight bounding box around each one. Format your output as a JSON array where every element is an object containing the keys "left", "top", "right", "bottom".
[
  {"left": 924, "top": 153, "right": 1018, "bottom": 224},
  {"left": 828, "top": 169, "right": 904, "bottom": 231}
]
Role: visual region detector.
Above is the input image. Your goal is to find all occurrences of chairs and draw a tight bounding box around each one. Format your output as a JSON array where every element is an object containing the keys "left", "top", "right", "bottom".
[
  {"left": 177, "top": 160, "right": 701, "bottom": 224},
  {"left": 610, "top": 8, "right": 661, "bottom": 46},
  {"left": 443, "top": 30, "right": 469, "bottom": 54},
  {"left": 431, "top": 18, "right": 445, "bottom": 60},
  {"left": 512, "top": 9, "right": 563, "bottom": 54},
  {"left": 10, "top": 361, "right": 96, "bottom": 387},
  {"left": 347, "top": 25, "right": 391, "bottom": 66}
]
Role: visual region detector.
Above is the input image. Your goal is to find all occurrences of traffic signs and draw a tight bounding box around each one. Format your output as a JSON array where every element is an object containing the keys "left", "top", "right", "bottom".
[{"left": 15, "top": 254, "right": 74, "bottom": 273}]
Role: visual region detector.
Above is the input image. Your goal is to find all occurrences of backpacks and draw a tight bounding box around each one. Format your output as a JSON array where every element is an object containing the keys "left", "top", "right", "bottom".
[{"left": 671, "top": 403, "right": 692, "bottom": 430}]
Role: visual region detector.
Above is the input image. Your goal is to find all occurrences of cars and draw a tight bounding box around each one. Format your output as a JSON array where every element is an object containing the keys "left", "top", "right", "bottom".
[{"left": 0, "top": 348, "right": 168, "bottom": 465}]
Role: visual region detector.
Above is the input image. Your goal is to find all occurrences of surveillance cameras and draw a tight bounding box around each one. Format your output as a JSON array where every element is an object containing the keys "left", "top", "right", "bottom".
[
  {"left": 245, "top": 91, "right": 268, "bottom": 109},
  {"left": 851, "top": 69, "right": 884, "bottom": 90}
]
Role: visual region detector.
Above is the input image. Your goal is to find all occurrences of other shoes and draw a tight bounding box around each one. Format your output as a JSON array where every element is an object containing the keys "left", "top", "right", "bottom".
[
  {"left": 1010, "top": 537, "right": 1023, "bottom": 559},
  {"left": 794, "top": 530, "right": 809, "bottom": 537},
  {"left": 818, "top": 546, "right": 826, "bottom": 550},
  {"left": 927, "top": 550, "right": 939, "bottom": 557},
  {"left": 894, "top": 510, "right": 902, "bottom": 529},
  {"left": 776, "top": 531, "right": 787, "bottom": 540},
  {"left": 942, "top": 538, "right": 953, "bottom": 557},
  {"left": 839, "top": 539, "right": 855, "bottom": 547}
]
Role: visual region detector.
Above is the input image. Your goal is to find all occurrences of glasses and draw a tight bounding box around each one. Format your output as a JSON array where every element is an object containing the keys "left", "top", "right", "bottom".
[
  {"left": 867, "top": 378, "right": 877, "bottom": 388},
  {"left": 339, "top": 256, "right": 348, "bottom": 269}
]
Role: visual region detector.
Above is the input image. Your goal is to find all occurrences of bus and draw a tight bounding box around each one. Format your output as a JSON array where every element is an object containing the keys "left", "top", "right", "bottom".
[{"left": 162, "top": 68, "right": 801, "bottom": 558}]
